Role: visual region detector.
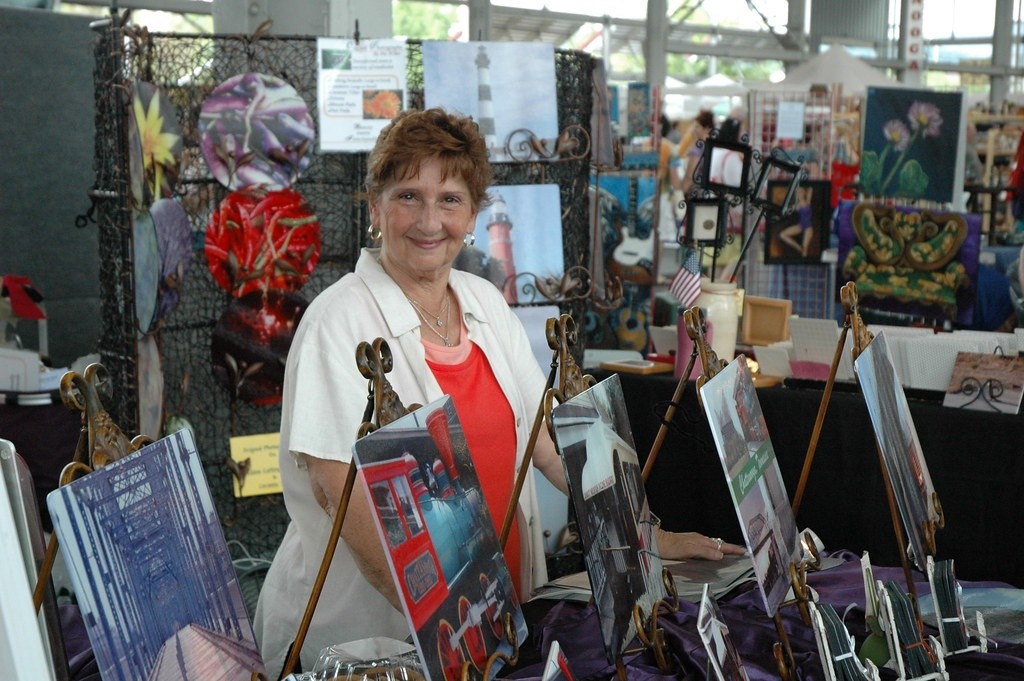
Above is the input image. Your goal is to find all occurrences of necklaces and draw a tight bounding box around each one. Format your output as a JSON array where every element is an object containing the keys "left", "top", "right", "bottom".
[{"left": 405, "top": 294, "right": 453, "bottom": 348}]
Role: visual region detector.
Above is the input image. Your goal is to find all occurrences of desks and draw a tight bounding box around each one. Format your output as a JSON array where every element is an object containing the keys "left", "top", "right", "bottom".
[
  {"left": 497, "top": 552, "right": 1024, "bottom": 681},
  {"left": 588, "top": 367, "right": 1024, "bottom": 589}
]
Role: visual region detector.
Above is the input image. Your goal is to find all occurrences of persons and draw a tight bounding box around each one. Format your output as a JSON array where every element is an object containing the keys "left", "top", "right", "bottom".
[
  {"left": 680, "top": 109, "right": 714, "bottom": 192},
  {"left": 252, "top": 107, "right": 746, "bottom": 680}
]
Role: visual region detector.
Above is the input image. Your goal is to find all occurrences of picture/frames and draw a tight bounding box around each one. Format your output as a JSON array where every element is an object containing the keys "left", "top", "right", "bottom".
[
  {"left": 764, "top": 179, "right": 831, "bottom": 265},
  {"left": 685, "top": 198, "right": 729, "bottom": 247},
  {"left": 741, "top": 295, "right": 792, "bottom": 345},
  {"left": 700, "top": 138, "right": 751, "bottom": 197}
]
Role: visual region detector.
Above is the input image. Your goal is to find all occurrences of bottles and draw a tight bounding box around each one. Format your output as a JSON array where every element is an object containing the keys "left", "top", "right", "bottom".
[{"left": 400, "top": 407, "right": 488, "bottom": 587}]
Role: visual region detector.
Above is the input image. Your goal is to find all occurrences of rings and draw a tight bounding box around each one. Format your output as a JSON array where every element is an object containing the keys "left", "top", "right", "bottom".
[{"left": 712, "top": 537, "right": 724, "bottom": 550}]
